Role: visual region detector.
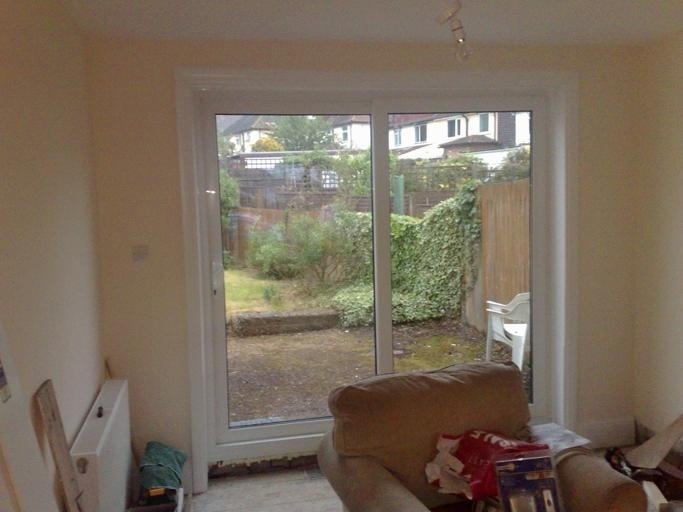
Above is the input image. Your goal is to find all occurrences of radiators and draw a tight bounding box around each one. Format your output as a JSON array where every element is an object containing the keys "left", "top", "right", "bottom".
[{"left": 68, "top": 377, "right": 133, "bottom": 512}]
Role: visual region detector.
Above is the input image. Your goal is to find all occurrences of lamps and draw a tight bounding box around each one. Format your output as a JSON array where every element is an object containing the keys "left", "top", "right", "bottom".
[{"left": 435, "top": 2, "right": 470, "bottom": 62}]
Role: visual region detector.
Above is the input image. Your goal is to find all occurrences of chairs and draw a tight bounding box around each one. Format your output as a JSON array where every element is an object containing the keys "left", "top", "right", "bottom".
[
  {"left": 484, "top": 293, "right": 531, "bottom": 370},
  {"left": 317, "top": 361, "right": 648, "bottom": 512}
]
